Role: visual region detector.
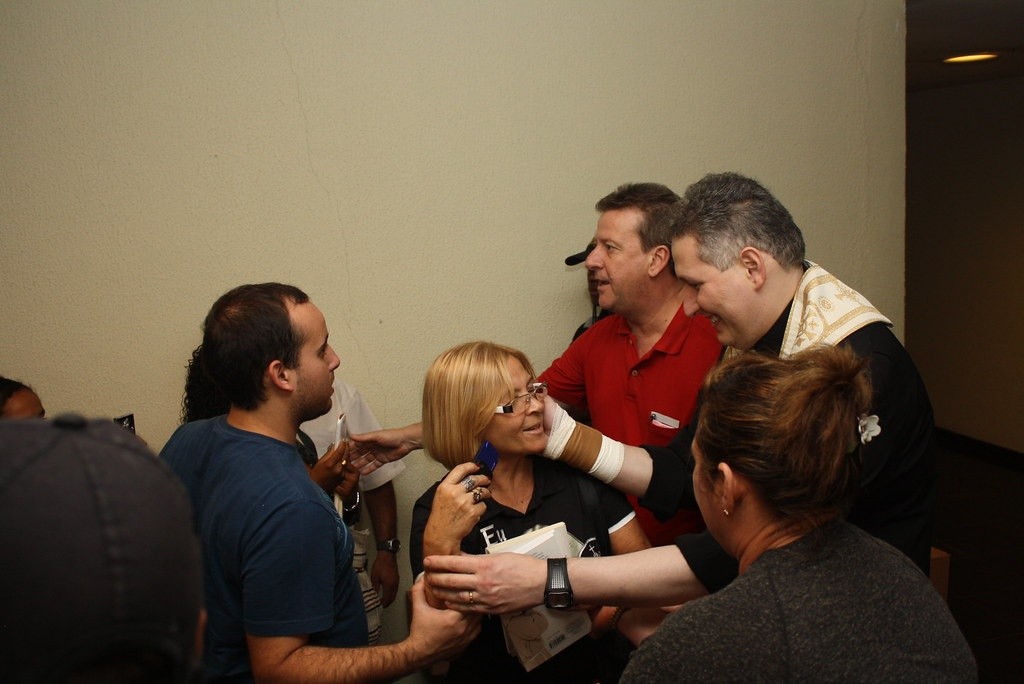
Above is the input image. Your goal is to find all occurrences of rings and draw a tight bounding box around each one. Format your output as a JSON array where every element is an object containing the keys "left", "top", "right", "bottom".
[
  {"left": 469, "top": 591, "right": 474, "bottom": 603},
  {"left": 339, "top": 459, "right": 347, "bottom": 466}
]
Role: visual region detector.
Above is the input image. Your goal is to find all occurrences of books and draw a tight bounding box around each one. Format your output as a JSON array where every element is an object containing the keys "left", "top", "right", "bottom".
[{"left": 485, "top": 522, "right": 592, "bottom": 671}]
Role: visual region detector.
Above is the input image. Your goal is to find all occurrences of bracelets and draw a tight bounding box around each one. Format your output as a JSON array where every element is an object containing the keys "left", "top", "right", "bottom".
[
  {"left": 608, "top": 607, "right": 628, "bottom": 630},
  {"left": 344, "top": 491, "right": 359, "bottom": 511}
]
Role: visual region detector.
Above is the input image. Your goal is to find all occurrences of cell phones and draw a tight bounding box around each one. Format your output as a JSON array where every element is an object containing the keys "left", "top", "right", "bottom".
[
  {"left": 465, "top": 441, "right": 498, "bottom": 488},
  {"left": 335, "top": 413, "right": 346, "bottom": 451}
]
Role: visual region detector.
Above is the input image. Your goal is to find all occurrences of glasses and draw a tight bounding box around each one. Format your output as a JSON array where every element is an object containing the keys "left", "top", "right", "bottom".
[{"left": 494, "top": 379, "right": 548, "bottom": 414}]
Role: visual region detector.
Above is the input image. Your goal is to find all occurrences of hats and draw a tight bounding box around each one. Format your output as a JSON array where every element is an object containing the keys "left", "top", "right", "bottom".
[
  {"left": 565, "top": 237, "right": 598, "bottom": 266},
  {"left": 0, "top": 410, "right": 203, "bottom": 684}
]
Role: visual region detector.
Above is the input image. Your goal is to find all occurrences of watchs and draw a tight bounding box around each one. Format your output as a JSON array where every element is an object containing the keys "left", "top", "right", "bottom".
[
  {"left": 544, "top": 558, "right": 574, "bottom": 610},
  {"left": 377, "top": 539, "right": 402, "bottom": 553}
]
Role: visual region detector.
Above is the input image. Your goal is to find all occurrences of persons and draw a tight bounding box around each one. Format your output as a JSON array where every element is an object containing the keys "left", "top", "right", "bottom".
[
  {"left": 409, "top": 341, "right": 653, "bottom": 684},
  {"left": 296, "top": 380, "right": 406, "bottom": 646},
  {"left": 348, "top": 183, "right": 723, "bottom": 547},
  {"left": 423, "top": 172, "right": 939, "bottom": 614},
  {"left": 179, "top": 342, "right": 362, "bottom": 526},
  {"left": 614, "top": 346, "right": 977, "bottom": 684},
  {"left": 157, "top": 283, "right": 481, "bottom": 684},
  {"left": 0, "top": 374, "right": 207, "bottom": 684}
]
